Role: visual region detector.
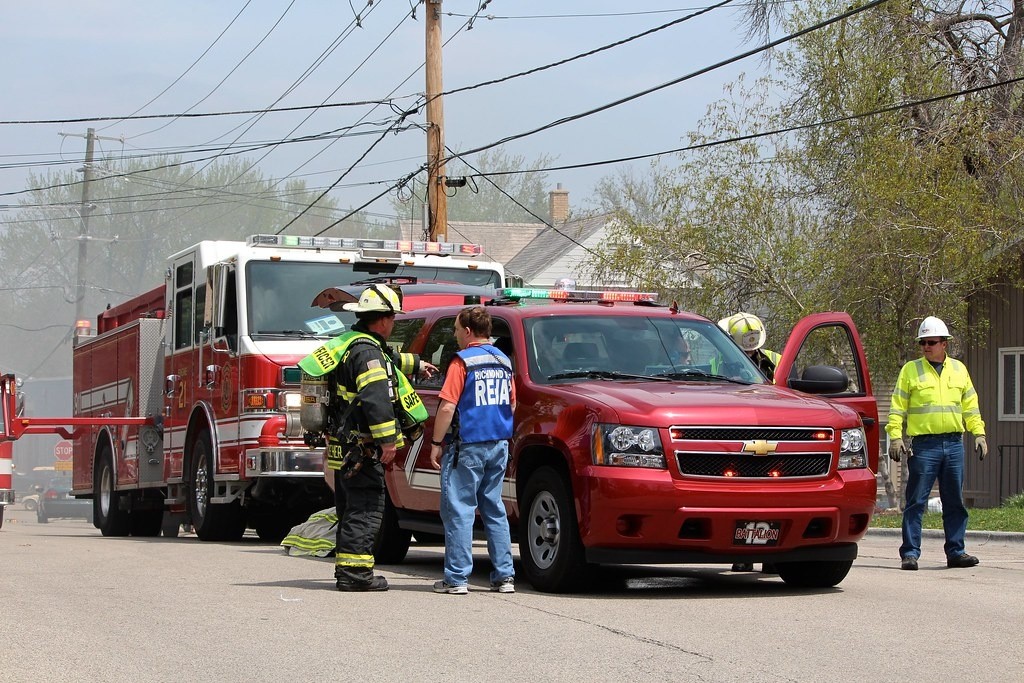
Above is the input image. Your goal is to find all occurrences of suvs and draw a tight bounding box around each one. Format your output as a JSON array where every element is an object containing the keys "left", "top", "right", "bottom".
[{"left": 323, "top": 278, "right": 879, "bottom": 593}]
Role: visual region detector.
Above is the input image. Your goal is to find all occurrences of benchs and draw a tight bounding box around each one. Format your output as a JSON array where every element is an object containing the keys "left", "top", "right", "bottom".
[{"left": 929, "top": 488, "right": 992, "bottom": 508}]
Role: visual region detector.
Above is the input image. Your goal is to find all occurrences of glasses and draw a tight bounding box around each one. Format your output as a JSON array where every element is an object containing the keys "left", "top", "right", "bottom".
[
  {"left": 674, "top": 352, "right": 691, "bottom": 358},
  {"left": 919, "top": 340, "right": 947, "bottom": 346}
]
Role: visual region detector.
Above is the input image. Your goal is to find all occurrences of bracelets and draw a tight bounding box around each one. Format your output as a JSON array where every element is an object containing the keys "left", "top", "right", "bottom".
[{"left": 431, "top": 440, "right": 443, "bottom": 446}]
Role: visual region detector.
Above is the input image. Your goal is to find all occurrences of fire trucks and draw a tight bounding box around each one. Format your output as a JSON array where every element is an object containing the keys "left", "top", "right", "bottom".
[{"left": 0, "top": 235, "right": 523, "bottom": 542}]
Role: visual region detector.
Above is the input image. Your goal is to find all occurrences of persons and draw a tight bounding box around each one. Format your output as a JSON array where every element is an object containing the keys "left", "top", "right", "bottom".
[
  {"left": 884, "top": 315, "right": 989, "bottom": 569},
  {"left": 658, "top": 336, "right": 693, "bottom": 368},
  {"left": 428, "top": 305, "right": 519, "bottom": 594},
  {"left": 706, "top": 312, "right": 798, "bottom": 384},
  {"left": 326, "top": 282, "right": 441, "bottom": 593}
]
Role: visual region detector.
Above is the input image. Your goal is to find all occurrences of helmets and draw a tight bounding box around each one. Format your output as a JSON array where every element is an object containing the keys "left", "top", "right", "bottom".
[
  {"left": 717, "top": 312, "right": 766, "bottom": 351},
  {"left": 914, "top": 315, "right": 954, "bottom": 341},
  {"left": 342, "top": 283, "right": 406, "bottom": 314}
]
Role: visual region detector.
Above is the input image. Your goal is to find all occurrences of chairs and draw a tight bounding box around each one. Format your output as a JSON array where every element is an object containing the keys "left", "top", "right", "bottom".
[
  {"left": 562, "top": 342, "right": 605, "bottom": 370},
  {"left": 605, "top": 342, "right": 656, "bottom": 370}
]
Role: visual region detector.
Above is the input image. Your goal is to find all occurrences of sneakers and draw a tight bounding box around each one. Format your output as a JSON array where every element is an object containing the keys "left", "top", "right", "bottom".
[
  {"left": 947, "top": 553, "right": 979, "bottom": 568},
  {"left": 490, "top": 576, "right": 514, "bottom": 592},
  {"left": 433, "top": 579, "right": 468, "bottom": 594},
  {"left": 902, "top": 556, "right": 918, "bottom": 570}
]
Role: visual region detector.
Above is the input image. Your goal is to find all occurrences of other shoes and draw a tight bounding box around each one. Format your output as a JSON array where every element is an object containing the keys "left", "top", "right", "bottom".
[{"left": 336, "top": 575, "right": 388, "bottom": 591}]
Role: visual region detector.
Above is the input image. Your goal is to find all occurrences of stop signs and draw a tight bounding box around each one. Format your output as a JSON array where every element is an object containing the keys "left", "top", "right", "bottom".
[{"left": 54, "top": 440, "right": 73, "bottom": 461}]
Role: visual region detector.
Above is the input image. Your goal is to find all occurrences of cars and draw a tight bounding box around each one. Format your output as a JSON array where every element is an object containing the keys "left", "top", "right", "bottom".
[{"left": 22, "top": 477, "right": 93, "bottom": 523}]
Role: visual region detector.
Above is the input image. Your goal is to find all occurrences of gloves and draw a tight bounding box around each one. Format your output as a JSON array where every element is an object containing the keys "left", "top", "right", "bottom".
[
  {"left": 888, "top": 439, "right": 907, "bottom": 462},
  {"left": 974, "top": 436, "right": 987, "bottom": 461}
]
